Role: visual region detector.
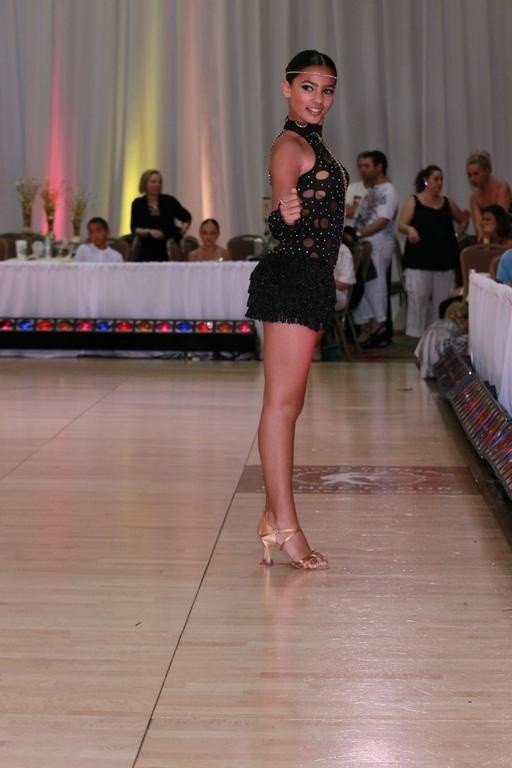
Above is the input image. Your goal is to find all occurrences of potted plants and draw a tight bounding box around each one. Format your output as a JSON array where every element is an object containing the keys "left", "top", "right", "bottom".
[
  {"left": 41, "top": 177, "right": 59, "bottom": 240},
  {"left": 71, "top": 193, "right": 89, "bottom": 244},
  {"left": 14, "top": 177, "right": 35, "bottom": 235}
]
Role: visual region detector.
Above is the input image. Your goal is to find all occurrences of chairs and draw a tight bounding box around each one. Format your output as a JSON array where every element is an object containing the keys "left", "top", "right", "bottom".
[
  {"left": 462, "top": 244, "right": 506, "bottom": 306},
  {"left": 133, "top": 236, "right": 139, "bottom": 253},
  {"left": 181, "top": 236, "right": 199, "bottom": 261},
  {"left": 168, "top": 239, "right": 181, "bottom": 261},
  {"left": 326, "top": 240, "right": 373, "bottom": 354},
  {"left": 227, "top": 234, "right": 265, "bottom": 260},
  {"left": 489, "top": 256, "right": 501, "bottom": 282},
  {"left": 87, "top": 238, "right": 128, "bottom": 262}
]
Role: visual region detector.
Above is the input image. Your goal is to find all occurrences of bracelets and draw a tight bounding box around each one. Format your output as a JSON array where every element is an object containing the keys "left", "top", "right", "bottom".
[
  {"left": 453, "top": 232, "right": 460, "bottom": 237},
  {"left": 356, "top": 231, "right": 363, "bottom": 238}
]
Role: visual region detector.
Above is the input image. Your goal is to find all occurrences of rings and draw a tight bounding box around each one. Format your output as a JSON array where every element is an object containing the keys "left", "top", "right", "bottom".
[{"left": 279, "top": 197, "right": 286, "bottom": 205}]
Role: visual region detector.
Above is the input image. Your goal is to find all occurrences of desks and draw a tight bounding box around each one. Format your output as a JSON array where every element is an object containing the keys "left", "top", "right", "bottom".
[
  {"left": 2, "top": 260, "right": 263, "bottom": 357},
  {"left": 467, "top": 274, "right": 512, "bottom": 417}
]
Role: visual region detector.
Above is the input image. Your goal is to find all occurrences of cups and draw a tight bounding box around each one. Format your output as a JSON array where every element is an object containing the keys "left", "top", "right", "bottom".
[{"left": 15, "top": 239, "right": 27, "bottom": 260}]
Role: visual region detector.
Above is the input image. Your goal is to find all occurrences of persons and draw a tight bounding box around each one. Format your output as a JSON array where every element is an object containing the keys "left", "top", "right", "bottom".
[
  {"left": 496, "top": 248, "right": 512, "bottom": 285},
  {"left": 130, "top": 170, "right": 192, "bottom": 262},
  {"left": 344, "top": 151, "right": 392, "bottom": 347},
  {"left": 187, "top": 218, "right": 231, "bottom": 260},
  {"left": 244, "top": 51, "right": 349, "bottom": 571},
  {"left": 74, "top": 217, "right": 124, "bottom": 262},
  {"left": 415, "top": 302, "right": 471, "bottom": 382},
  {"left": 307, "top": 242, "right": 357, "bottom": 361},
  {"left": 399, "top": 165, "right": 468, "bottom": 340},
  {"left": 456, "top": 149, "right": 512, "bottom": 287},
  {"left": 353, "top": 150, "right": 400, "bottom": 348},
  {"left": 439, "top": 204, "right": 511, "bottom": 318}
]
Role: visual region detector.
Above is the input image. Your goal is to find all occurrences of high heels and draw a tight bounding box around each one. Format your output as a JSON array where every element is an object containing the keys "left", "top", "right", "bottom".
[{"left": 256, "top": 514, "right": 329, "bottom": 571}]
